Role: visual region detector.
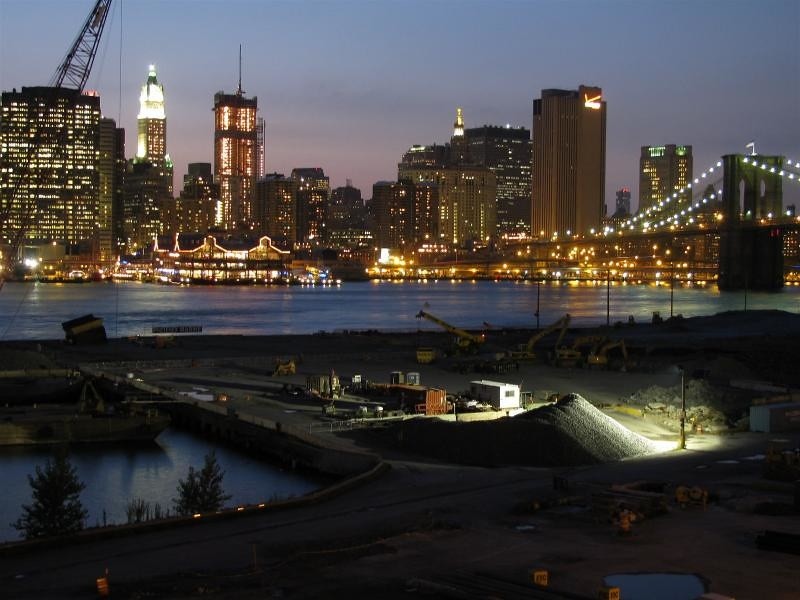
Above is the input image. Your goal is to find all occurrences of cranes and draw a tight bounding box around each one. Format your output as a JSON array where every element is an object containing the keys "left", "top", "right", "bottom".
[{"left": 0, "top": 0, "right": 114, "bottom": 287}]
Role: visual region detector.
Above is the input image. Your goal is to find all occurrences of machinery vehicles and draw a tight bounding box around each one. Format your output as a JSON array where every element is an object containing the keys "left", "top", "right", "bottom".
[
  {"left": 475, "top": 312, "right": 640, "bottom": 375},
  {"left": 413, "top": 310, "right": 494, "bottom": 376}
]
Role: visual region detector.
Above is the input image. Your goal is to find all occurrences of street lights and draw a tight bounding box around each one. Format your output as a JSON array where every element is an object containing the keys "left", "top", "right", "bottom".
[{"left": 674, "top": 362, "right": 686, "bottom": 449}]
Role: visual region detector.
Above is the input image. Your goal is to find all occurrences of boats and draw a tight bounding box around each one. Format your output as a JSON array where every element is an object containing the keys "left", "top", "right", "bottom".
[{"left": 0, "top": 371, "right": 174, "bottom": 447}]
